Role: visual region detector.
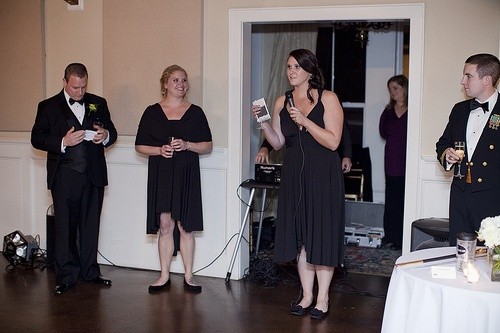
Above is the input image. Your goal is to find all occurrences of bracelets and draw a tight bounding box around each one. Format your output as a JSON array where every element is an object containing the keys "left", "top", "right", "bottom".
[{"left": 187, "top": 141, "right": 191, "bottom": 151}]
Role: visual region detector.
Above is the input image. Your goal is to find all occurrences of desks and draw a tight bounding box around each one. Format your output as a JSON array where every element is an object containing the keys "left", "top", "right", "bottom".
[
  {"left": 225, "top": 182, "right": 279, "bottom": 283},
  {"left": 381, "top": 246, "right": 500, "bottom": 332}
]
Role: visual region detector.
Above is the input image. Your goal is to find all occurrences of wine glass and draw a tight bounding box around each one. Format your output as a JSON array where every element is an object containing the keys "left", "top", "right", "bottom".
[{"left": 454, "top": 141, "right": 466, "bottom": 178}]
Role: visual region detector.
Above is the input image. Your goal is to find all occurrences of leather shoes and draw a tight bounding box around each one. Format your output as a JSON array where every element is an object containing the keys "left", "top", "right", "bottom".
[
  {"left": 54, "top": 283, "right": 78, "bottom": 295},
  {"left": 80, "top": 273, "right": 112, "bottom": 285}
]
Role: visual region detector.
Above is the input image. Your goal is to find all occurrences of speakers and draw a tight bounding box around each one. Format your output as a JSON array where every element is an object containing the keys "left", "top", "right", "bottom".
[{"left": 411, "top": 217, "right": 450, "bottom": 252}]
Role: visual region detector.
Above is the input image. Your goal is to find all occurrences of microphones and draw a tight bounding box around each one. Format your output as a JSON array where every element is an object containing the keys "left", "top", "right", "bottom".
[{"left": 285, "top": 91, "right": 300, "bottom": 127}]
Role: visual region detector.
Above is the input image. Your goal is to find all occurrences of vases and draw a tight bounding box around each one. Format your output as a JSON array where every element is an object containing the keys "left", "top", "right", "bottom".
[{"left": 491, "top": 250, "right": 500, "bottom": 282}]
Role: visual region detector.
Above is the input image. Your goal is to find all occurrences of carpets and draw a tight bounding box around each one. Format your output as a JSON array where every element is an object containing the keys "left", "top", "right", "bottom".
[{"left": 331, "top": 244, "right": 402, "bottom": 298}]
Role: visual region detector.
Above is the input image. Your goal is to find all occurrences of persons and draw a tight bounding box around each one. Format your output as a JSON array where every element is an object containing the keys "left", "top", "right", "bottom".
[
  {"left": 379, "top": 75, "right": 408, "bottom": 250},
  {"left": 135, "top": 65, "right": 213, "bottom": 292},
  {"left": 437, "top": 54, "right": 500, "bottom": 247},
  {"left": 253, "top": 48, "right": 352, "bottom": 319},
  {"left": 30, "top": 64, "right": 118, "bottom": 295}
]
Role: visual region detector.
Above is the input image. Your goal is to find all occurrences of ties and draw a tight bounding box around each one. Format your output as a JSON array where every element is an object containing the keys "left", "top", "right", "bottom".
[{"left": 470, "top": 100, "right": 489, "bottom": 112}]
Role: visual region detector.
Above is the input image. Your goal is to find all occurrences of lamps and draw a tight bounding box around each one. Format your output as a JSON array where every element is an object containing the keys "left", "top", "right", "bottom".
[
  {"left": 3, "top": 230, "right": 39, "bottom": 268},
  {"left": 330, "top": 20, "right": 393, "bottom": 48}
]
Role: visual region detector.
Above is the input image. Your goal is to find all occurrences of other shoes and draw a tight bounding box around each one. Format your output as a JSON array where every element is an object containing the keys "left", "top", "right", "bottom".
[
  {"left": 290, "top": 299, "right": 315, "bottom": 316},
  {"left": 310, "top": 302, "right": 330, "bottom": 319},
  {"left": 148, "top": 276, "right": 171, "bottom": 294},
  {"left": 391, "top": 245, "right": 401, "bottom": 249},
  {"left": 183, "top": 277, "right": 202, "bottom": 293},
  {"left": 381, "top": 239, "right": 389, "bottom": 244}
]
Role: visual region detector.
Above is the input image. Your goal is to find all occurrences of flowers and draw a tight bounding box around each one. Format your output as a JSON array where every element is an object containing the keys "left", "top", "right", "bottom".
[
  {"left": 86, "top": 103, "right": 101, "bottom": 116},
  {"left": 475, "top": 215, "right": 500, "bottom": 273}
]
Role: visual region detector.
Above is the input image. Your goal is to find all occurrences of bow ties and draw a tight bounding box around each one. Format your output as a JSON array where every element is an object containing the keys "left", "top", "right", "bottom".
[{"left": 69, "top": 97, "right": 85, "bottom": 106}]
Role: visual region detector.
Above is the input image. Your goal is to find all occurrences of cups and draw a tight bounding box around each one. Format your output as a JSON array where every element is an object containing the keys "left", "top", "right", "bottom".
[
  {"left": 455, "top": 232, "right": 477, "bottom": 272},
  {"left": 488, "top": 247, "right": 500, "bottom": 283},
  {"left": 92, "top": 117, "right": 104, "bottom": 142},
  {"left": 167, "top": 136, "right": 178, "bottom": 158}
]
